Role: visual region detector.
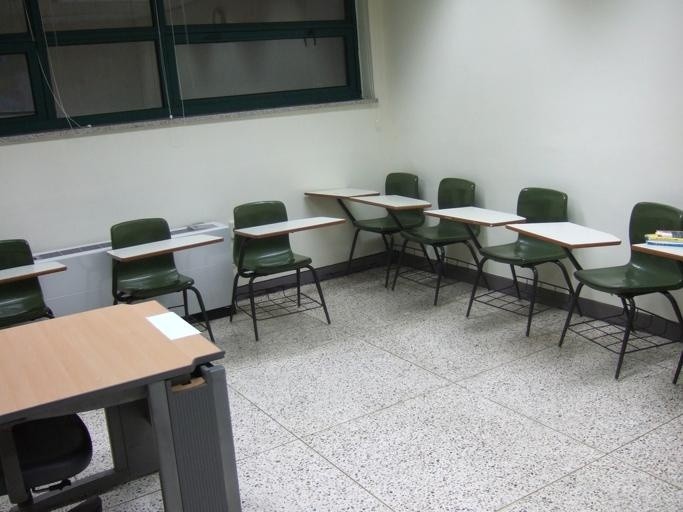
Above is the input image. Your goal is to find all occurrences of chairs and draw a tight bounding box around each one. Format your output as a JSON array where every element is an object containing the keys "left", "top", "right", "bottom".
[
  {"left": 0, "top": 240, "right": 55, "bottom": 328},
  {"left": 232, "top": 200, "right": 331, "bottom": 341},
  {"left": 111, "top": 219, "right": 217, "bottom": 345},
  {"left": 1, "top": 413, "right": 92, "bottom": 495}
]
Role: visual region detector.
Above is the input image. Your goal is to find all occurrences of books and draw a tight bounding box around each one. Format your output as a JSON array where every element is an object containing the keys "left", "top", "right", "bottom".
[{"left": 644, "top": 230, "right": 683, "bottom": 247}]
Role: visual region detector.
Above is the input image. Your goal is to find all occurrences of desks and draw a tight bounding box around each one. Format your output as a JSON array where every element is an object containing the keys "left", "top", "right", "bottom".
[
  {"left": 106, "top": 234, "right": 223, "bottom": 302},
  {"left": 234, "top": 215, "right": 346, "bottom": 277},
  {"left": 0, "top": 301, "right": 243, "bottom": 512},
  {"left": 0, "top": 261, "right": 67, "bottom": 285}
]
